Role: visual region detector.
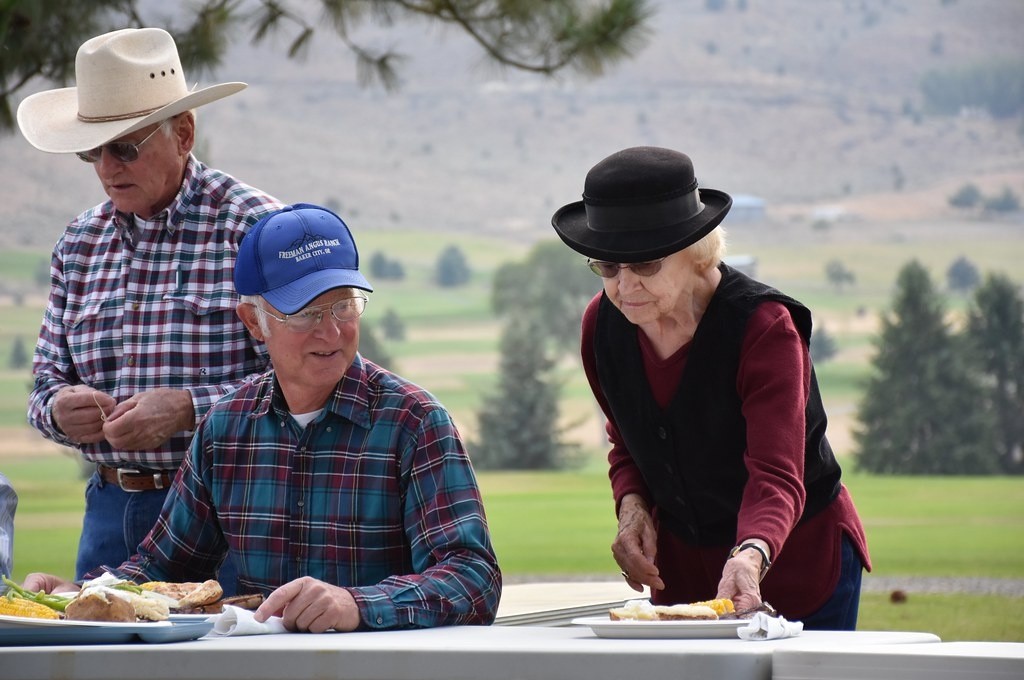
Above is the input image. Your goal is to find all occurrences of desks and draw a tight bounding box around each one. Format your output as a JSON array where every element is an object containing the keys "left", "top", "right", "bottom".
[
  {"left": 771, "top": 642, "right": 1024, "bottom": 680},
  {"left": 0, "top": 623, "right": 943, "bottom": 680}
]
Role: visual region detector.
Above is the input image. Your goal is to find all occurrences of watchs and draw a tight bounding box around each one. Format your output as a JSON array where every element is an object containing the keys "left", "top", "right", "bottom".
[{"left": 728, "top": 543, "right": 771, "bottom": 582}]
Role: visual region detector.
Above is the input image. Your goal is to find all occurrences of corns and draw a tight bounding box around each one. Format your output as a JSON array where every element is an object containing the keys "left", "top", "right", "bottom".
[
  {"left": 688, "top": 598, "right": 734, "bottom": 615},
  {"left": 0, "top": 596, "right": 60, "bottom": 620}
]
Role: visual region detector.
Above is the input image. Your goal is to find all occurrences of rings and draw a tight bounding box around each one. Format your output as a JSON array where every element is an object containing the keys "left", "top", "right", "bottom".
[{"left": 621, "top": 570, "right": 629, "bottom": 579}]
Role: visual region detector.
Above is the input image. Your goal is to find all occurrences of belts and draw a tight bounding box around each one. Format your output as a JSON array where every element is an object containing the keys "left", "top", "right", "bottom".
[{"left": 96, "top": 462, "right": 180, "bottom": 492}]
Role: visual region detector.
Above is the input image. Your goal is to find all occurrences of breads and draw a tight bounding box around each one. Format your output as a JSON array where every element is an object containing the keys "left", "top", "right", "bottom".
[
  {"left": 125, "top": 578, "right": 224, "bottom": 622},
  {"left": 605, "top": 597, "right": 719, "bottom": 620}
]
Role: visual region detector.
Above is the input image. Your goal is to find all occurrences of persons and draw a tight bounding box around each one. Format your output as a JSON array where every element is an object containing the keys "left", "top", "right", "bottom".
[
  {"left": 16, "top": 28, "right": 288, "bottom": 590},
  {"left": 20, "top": 204, "right": 503, "bottom": 635},
  {"left": 552, "top": 146, "right": 872, "bottom": 631}
]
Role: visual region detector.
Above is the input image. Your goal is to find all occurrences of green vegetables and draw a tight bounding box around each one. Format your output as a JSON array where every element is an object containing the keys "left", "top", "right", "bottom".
[{"left": 2, "top": 575, "right": 143, "bottom": 613}]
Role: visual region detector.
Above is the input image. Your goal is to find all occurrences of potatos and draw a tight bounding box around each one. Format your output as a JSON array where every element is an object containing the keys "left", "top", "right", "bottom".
[{"left": 63, "top": 585, "right": 137, "bottom": 622}]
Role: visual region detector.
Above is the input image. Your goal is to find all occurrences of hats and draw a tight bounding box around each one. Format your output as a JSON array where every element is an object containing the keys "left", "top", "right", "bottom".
[
  {"left": 233, "top": 203, "right": 374, "bottom": 316},
  {"left": 550, "top": 146, "right": 733, "bottom": 263},
  {"left": 16, "top": 28, "right": 250, "bottom": 152}
]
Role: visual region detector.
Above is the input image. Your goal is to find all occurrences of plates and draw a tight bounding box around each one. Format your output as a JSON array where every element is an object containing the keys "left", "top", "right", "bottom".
[
  {"left": 572, "top": 613, "right": 751, "bottom": 639},
  {"left": 167, "top": 613, "right": 218, "bottom": 622},
  {"left": 1, "top": 621, "right": 217, "bottom": 647}
]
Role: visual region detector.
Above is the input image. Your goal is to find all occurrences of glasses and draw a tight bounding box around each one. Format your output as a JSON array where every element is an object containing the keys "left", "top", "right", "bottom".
[
  {"left": 586, "top": 257, "right": 669, "bottom": 279},
  {"left": 253, "top": 290, "right": 369, "bottom": 332},
  {"left": 76, "top": 116, "right": 176, "bottom": 162}
]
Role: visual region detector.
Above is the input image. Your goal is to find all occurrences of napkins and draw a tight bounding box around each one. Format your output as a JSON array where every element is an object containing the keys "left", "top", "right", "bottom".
[
  {"left": 736, "top": 612, "right": 805, "bottom": 640},
  {"left": 197, "top": 603, "right": 291, "bottom": 638}
]
